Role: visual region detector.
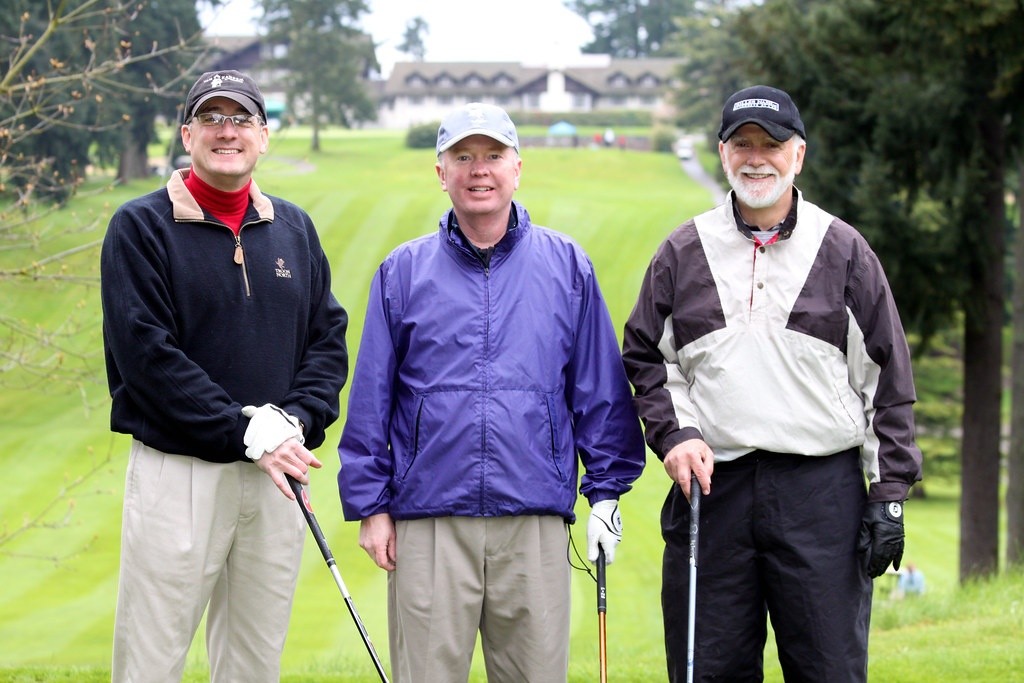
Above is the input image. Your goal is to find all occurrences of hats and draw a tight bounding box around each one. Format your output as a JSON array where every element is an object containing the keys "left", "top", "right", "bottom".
[
  {"left": 436, "top": 103, "right": 521, "bottom": 156},
  {"left": 719, "top": 84, "right": 807, "bottom": 143},
  {"left": 183, "top": 69, "right": 267, "bottom": 125}
]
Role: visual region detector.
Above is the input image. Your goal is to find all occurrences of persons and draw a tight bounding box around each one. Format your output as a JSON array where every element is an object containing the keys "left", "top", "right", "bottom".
[
  {"left": 624, "top": 85, "right": 921, "bottom": 683},
  {"left": 336, "top": 103, "right": 648, "bottom": 683},
  {"left": 99, "top": 69, "right": 349, "bottom": 682}
]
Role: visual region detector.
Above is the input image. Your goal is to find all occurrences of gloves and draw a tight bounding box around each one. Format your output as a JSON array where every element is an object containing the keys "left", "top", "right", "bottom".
[
  {"left": 587, "top": 498, "right": 624, "bottom": 566},
  {"left": 241, "top": 403, "right": 306, "bottom": 460},
  {"left": 858, "top": 500, "right": 905, "bottom": 579}
]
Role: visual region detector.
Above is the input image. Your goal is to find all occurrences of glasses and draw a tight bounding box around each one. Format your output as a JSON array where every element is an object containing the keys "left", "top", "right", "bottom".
[{"left": 192, "top": 113, "right": 265, "bottom": 128}]
